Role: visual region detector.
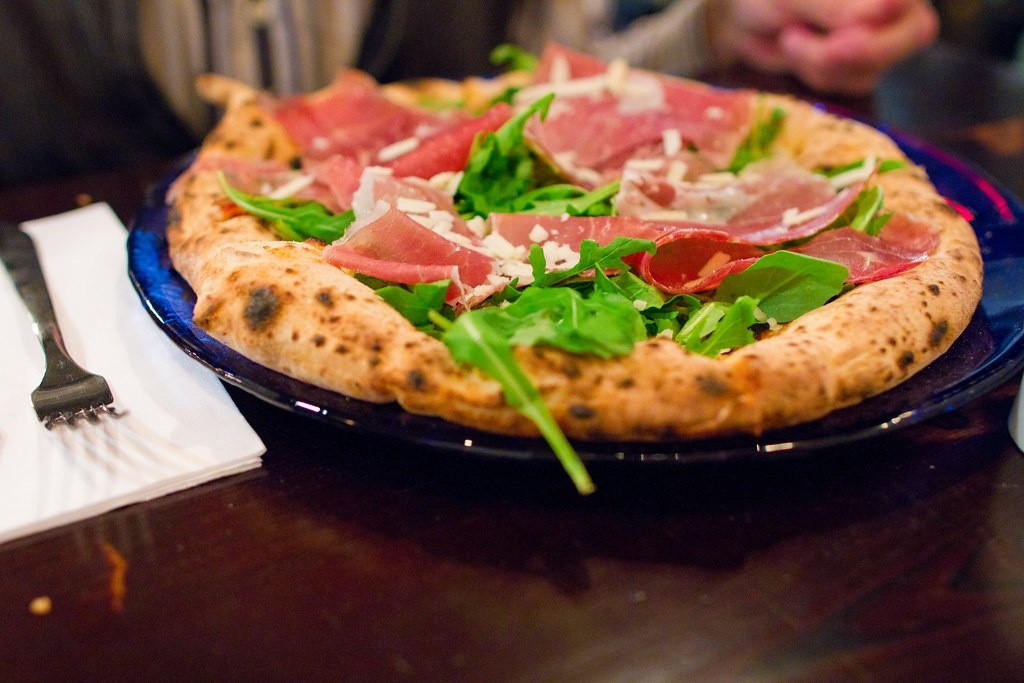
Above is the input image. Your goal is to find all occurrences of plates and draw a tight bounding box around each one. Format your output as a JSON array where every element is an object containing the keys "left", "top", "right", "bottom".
[{"left": 129, "top": 120, "right": 1022, "bottom": 465}]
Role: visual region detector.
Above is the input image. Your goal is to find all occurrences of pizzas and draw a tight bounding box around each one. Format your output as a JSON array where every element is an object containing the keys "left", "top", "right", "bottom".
[{"left": 160, "top": 39, "right": 985, "bottom": 496}]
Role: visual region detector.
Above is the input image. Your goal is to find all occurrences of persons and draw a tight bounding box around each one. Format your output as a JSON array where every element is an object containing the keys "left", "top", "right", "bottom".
[
  {"left": 0, "top": 0, "right": 504, "bottom": 177},
  {"left": 510, "top": 0, "right": 943, "bottom": 99}
]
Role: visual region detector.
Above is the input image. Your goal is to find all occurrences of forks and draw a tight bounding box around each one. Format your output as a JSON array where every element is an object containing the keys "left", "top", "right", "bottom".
[{"left": 1, "top": 217, "right": 124, "bottom": 431}]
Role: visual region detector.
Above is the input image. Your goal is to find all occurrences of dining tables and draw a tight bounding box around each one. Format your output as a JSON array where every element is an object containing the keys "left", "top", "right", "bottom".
[{"left": 0, "top": 80, "right": 1024, "bottom": 683}]
